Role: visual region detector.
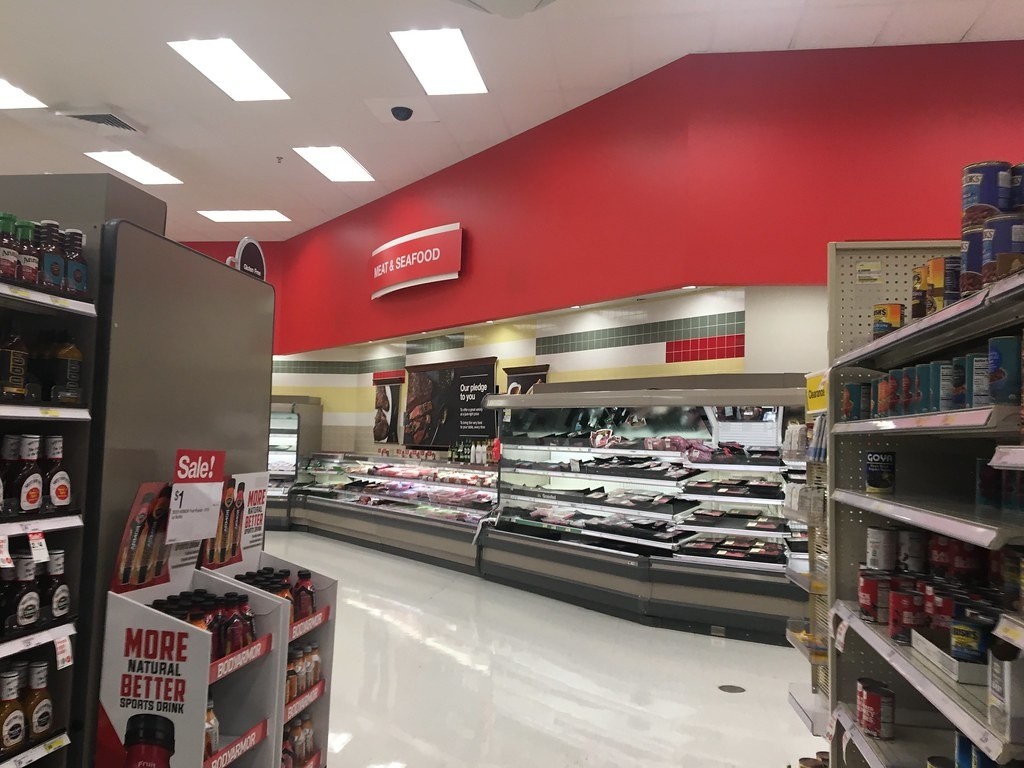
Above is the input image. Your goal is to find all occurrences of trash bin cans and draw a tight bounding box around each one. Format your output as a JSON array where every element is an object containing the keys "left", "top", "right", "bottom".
[{"left": 288, "top": 489, "right": 311, "bottom": 532}]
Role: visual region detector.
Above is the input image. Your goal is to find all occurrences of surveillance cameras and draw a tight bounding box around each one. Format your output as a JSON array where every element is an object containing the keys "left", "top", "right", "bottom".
[{"left": 391, "top": 107, "right": 413, "bottom": 121}]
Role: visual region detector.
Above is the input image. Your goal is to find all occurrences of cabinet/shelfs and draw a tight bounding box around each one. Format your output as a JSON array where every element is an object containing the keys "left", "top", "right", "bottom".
[
  {"left": 264, "top": 395, "right": 325, "bottom": 531},
  {"left": 0, "top": 274, "right": 97, "bottom": 767},
  {"left": 290, "top": 453, "right": 499, "bottom": 576},
  {"left": 98, "top": 551, "right": 338, "bottom": 768},
  {"left": 480, "top": 389, "right": 808, "bottom": 635}
]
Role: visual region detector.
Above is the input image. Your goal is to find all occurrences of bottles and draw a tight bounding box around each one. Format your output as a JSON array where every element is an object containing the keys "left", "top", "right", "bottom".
[
  {"left": 0, "top": 549, "right": 70, "bottom": 634},
  {"left": 118, "top": 486, "right": 170, "bottom": 586},
  {"left": 0, "top": 433, "right": 70, "bottom": 516},
  {"left": 447, "top": 438, "right": 493, "bottom": 464},
  {"left": 147, "top": 568, "right": 320, "bottom": 768},
  {"left": 207, "top": 479, "right": 245, "bottom": 564},
  {"left": 0, "top": 660, "right": 54, "bottom": 758},
  {"left": 0, "top": 211, "right": 90, "bottom": 294},
  {"left": 0, "top": 319, "right": 83, "bottom": 407},
  {"left": 124, "top": 714, "right": 175, "bottom": 768},
  {"left": 526, "top": 379, "right": 541, "bottom": 394}
]
